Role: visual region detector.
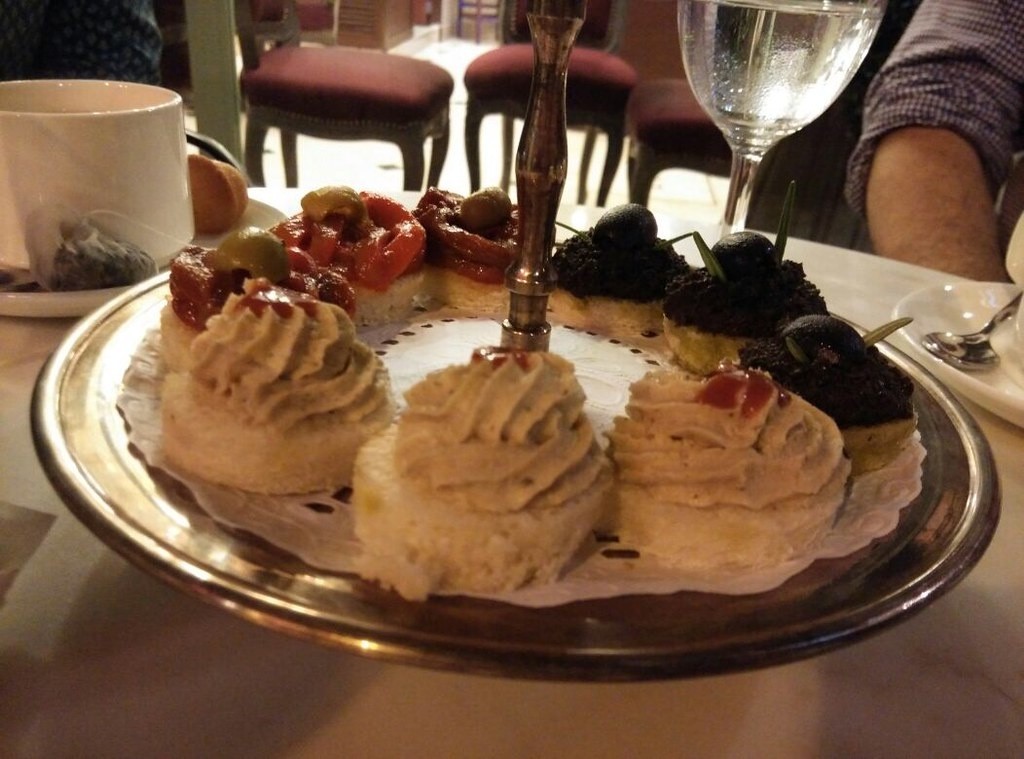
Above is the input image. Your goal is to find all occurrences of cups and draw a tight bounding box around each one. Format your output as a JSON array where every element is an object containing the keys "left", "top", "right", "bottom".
[{"left": 0, "top": 77, "right": 196, "bottom": 292}]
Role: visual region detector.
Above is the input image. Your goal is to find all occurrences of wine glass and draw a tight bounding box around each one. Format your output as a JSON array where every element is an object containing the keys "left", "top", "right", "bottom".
[{"left": 674, "top": 0, "right": 886, "bottom": 245}]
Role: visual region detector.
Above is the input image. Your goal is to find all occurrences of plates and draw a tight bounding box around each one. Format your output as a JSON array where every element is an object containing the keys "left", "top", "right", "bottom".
[
  {"left": 890, "top": 282, "right": 1024, "bottom": 431},
  {"left": 28, "top": 272, "right": 1002, "bottom": 686},
  {"left": 0, "top": 199, "right": 292, "bottom": 320}
]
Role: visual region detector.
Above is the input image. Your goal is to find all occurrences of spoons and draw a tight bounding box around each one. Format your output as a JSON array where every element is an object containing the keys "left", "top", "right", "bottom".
[{"left": 920, "top": 290, "right": 1024, "bottom": 371}]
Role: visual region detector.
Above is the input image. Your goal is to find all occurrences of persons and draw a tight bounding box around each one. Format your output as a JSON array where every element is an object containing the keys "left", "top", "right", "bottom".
[
  {"left": 844, "top": 0, "right": 1024, "bottom": 283},
  {"left": 0, "top": 0, "right": 164, "bottom": 87}
]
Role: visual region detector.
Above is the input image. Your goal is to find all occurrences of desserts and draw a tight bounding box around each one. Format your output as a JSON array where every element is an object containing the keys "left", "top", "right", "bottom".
[{"left": 165, "top": 181, "right": 914, "bottom": 602}]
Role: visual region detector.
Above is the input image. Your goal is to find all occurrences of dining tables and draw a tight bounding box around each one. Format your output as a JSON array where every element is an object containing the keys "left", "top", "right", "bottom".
[{"left": 0, "top": 190, "right": 1024, "bottom": 759}]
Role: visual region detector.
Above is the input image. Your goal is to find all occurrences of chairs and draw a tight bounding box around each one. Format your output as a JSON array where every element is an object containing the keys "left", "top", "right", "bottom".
[
  {"left": 233, "top": 0, "right": 455, "bottom": 191},
  {"left": 465, "top": 0, "right": 734, "bottom": 207}
]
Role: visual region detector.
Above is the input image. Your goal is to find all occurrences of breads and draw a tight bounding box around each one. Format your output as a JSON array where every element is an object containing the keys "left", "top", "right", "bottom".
[{"left": 187, "top": 155, "right": 249, "bottom": 235}]
